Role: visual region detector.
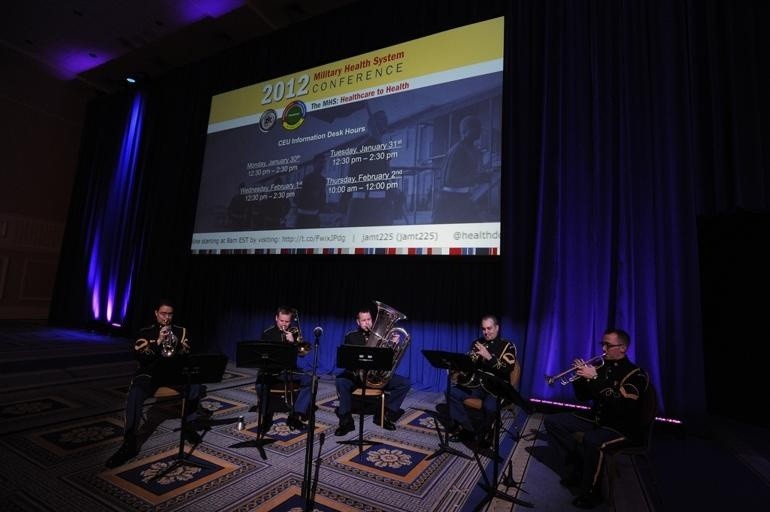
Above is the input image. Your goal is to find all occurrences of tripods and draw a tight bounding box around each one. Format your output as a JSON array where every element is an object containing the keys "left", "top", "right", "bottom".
[
  {"left": 474, "top": 398, "right": 534, "bottom": 511},
  {"left": 156, "top": 398, "right": 214, "bottom": 480},
  {"left": 426, "top": 370, "right": 474, "bottom": 461},
  {"left": 335, "top": 367, "right": 384, "bottom": 453},
  {"left": 228, "top": 373, "right": 277, "bottom": 459}
]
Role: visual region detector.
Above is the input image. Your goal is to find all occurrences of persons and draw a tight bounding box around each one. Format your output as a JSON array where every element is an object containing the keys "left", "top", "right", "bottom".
[
  {"left": 443, "top": 314, "right": 516, "bottom": 449},
  {"left": 226, "top": 153, "right": 328, "bottom": 230},
  {"left": 543, "top": 328, "right": 650, "bottom": 508},
  {"left": 335, "top": 305, "right": 411, "bottom": 436},
  {"left": 342, "top": 109, "right": 406, "bottom": 227},
  {"left": 255, "top": 306, "right": 318, "bottom": 431},
  {"left": 431, "top": 114, "right": 492, "bottom": 223},
  {"left": 104, "top": 298, "right": 204, "bottom": 469}
]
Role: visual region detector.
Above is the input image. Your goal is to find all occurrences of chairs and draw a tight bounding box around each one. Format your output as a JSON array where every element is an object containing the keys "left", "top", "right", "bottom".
[
  {"left": 453, "top": 355, "right": 522, "bottom": 447},
  {"left": 559, "top": 379, "right": 659, "bottom": 503}
]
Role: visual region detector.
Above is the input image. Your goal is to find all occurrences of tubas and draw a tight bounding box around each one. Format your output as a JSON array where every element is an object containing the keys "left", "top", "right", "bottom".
[{"left": 360, "top": 301, "right": 409, "bottom": 388}]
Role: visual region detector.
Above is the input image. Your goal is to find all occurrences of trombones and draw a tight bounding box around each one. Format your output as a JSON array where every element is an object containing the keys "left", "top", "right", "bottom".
[{"left": 280, "top": 310, "right": 312, "bottom": 408}]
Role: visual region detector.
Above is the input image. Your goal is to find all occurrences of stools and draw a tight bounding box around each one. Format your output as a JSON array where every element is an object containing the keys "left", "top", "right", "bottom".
[
  {"left": 123, "top": 372, "right": 190, "bottom": 462},
  {"left": 349, "top": 385, "right": 386, "bottom": 430},
  {"left": 255, "top": 371, "right": 314, "bottom": 431}
]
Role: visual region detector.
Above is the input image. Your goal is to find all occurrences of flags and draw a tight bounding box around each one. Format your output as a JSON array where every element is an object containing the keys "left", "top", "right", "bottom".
[{"left": 310, "top": 98, "right": 368, "bottom": 123}]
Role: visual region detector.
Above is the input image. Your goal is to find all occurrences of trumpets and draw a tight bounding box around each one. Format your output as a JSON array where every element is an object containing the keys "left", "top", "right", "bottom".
[
  {"left": 544, "top": 353, "right": 607, "bottom": 386},
  {"left": 161, "top": 319, "right": 175, "bottom": 357}
]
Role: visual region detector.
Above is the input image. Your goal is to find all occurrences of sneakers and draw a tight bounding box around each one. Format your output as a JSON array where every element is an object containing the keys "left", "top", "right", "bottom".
[
  {"left": 286, "top": 416, "right": 306, "bottom": 430},
  {"left": 107, "top": 443, "right": 135, "bottom": 467},
  {"left": 574, "top": 491, "right": 605, "bottom": 509},
  {"left": 184, "top": 433, "right": 203, "bottom": 445},
  {"left": 451, "top": 431, "right": 466, "bottom": 442},
  {"left": 373, "top": 416, "right": 396, "bottom": 430},
  {"left": 261, "top": 419, "right": 273, "bottom": 432},
  {"left": 335, "top": 424, "right": 355, "bottom": 436}
]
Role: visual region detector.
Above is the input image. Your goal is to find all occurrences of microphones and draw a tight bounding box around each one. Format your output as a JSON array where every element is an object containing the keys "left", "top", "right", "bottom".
[{"left": 314, "top": 327, "right": 323, "bottom": 344}]
